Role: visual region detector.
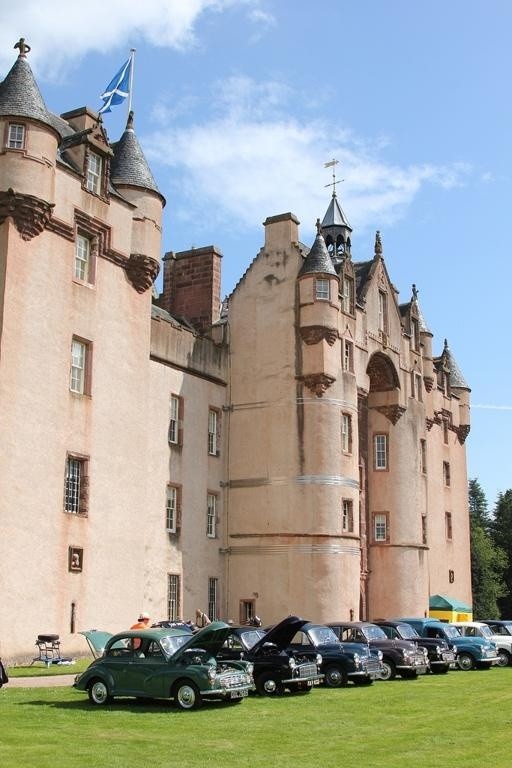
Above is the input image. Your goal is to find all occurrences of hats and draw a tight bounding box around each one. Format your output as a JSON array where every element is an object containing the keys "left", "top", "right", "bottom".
[{"left": 138, "top": 612, "right": 150, "bottom": 619}]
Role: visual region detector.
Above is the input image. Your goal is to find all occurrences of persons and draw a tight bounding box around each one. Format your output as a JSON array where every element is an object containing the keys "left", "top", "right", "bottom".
[
  {"left": 128, "top": 612, "right": 152, "bottom": 650},
  {"left": 193, "top": 608, "right": 212, "bottom": 629}
]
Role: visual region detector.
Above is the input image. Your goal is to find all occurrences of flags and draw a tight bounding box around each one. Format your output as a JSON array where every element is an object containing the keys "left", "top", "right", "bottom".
[{"left": 97, "top": 56, "right": 133, "bottom": 116}]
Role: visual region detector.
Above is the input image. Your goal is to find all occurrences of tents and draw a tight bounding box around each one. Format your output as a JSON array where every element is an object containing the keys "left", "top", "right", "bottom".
[{"left": 429, "top": 594, "right": 474, "bottom": 626}]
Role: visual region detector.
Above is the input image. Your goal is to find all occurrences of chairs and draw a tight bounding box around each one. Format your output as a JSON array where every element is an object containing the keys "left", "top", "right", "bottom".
[{"left": 120, "top": 650, "right": 145, "bottom": 658}]
